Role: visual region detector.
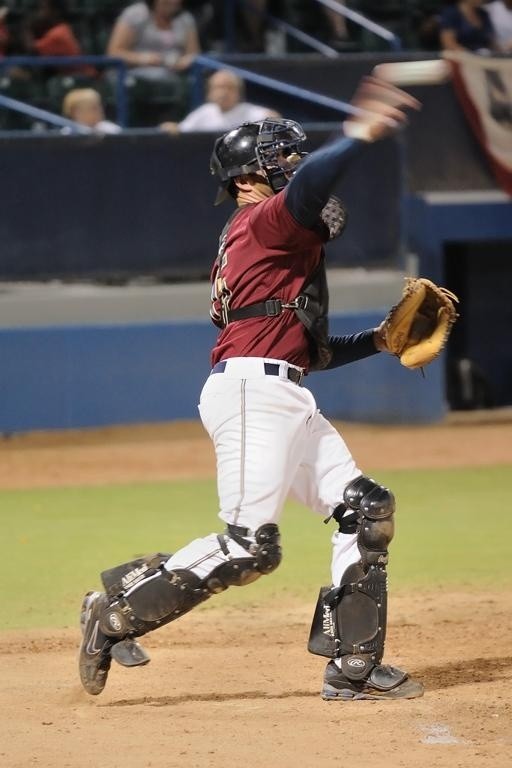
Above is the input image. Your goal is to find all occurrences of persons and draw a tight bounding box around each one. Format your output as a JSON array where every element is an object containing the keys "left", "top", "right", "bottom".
[
  {"left": 1, "top": 0, "right": 283, "bottom": 136},
  {"left": 421, "top": 1, "right": 511, "bottom": 56},
  {"left": 242, "top": 0, "right": 356, "bottom": 56},
  {"left": 74, "top": 71, "right": 458, "bottom": 702}
]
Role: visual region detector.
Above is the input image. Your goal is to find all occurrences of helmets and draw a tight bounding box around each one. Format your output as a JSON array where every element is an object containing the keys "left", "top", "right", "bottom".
[{"left": 209, "top": 117, "right": 310, "bottom": 207}]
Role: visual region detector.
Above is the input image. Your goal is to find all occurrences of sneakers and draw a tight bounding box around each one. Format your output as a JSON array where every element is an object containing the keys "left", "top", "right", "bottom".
[
  {"left": 79, "top": 590, "right": 116, "bottom": 694},
  {"left": 321, "top": 659, "right": 424, "bottom": 703}
]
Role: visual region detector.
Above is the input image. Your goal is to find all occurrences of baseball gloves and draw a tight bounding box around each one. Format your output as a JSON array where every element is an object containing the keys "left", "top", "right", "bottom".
[{"left": 375, "top": 277, "right": 460, "bottom": 378}]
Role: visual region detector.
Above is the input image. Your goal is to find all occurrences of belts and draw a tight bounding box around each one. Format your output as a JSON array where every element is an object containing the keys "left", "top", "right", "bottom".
[{"left": 211, "top": 360, "right": 303, "bottom": 385}]
